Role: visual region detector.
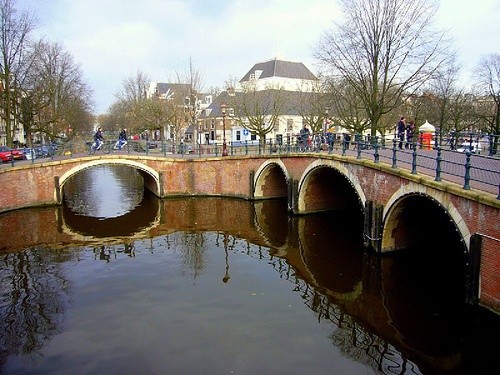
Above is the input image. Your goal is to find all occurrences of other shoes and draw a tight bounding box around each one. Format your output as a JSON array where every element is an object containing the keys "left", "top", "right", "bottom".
[
  {"left": 398, "top": 146, "right": 404, "bottom": 150},
  {"left": 410, "top": 148, "right": 414, "bottom": 150},
  {"left": 405, "top": 146, "right": 409, "bottom": 149}
]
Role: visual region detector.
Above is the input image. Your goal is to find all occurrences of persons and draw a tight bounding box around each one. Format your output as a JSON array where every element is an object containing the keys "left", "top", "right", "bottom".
[
  {"left": 113, "top": 127, "right": 130, "bottom": 150},
  {"left": 405, "top": 120, "right": 416, "bottom": 150},
  {"left": 300, "top": 124, "right": 314, "bottom": 146},
  {"left": 90, "top": 127, "right": 105, "bottom": 151},
  {"left": 398, "top": 116, "right": 409, "bottom": 150}
]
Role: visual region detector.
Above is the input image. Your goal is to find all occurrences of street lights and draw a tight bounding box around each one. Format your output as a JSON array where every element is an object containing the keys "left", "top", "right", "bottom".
[
  {"left": 323, "top": 107, "right": 330, "bottom": 135},
  {"left": 219, "top": 103, "right": 229, "bottom": 157}
]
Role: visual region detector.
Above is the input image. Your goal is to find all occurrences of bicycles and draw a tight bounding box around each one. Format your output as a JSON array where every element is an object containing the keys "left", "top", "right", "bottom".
[
  {"left": 111, "top": 140, "right": 133, "bottom": 153},
  {"left": 84, "top": 140, "right": 110, "bottom": 155}
]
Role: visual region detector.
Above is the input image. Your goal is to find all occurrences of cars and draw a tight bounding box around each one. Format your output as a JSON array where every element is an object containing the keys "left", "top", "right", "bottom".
[
  {"left": 0, "top": 146, "right": 13, "bottom": 163},
  {"left": 146, "top": 139, "right": 194, "bottom": 154},
  {"left": 12, "top": 143, "right": 62, "bottom": 160}
]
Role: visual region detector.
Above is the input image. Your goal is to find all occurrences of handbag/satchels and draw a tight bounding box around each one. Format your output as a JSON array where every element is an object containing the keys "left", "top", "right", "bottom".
[{"left": 398, "top": 132, "right": 405, "bottom": 137}]
